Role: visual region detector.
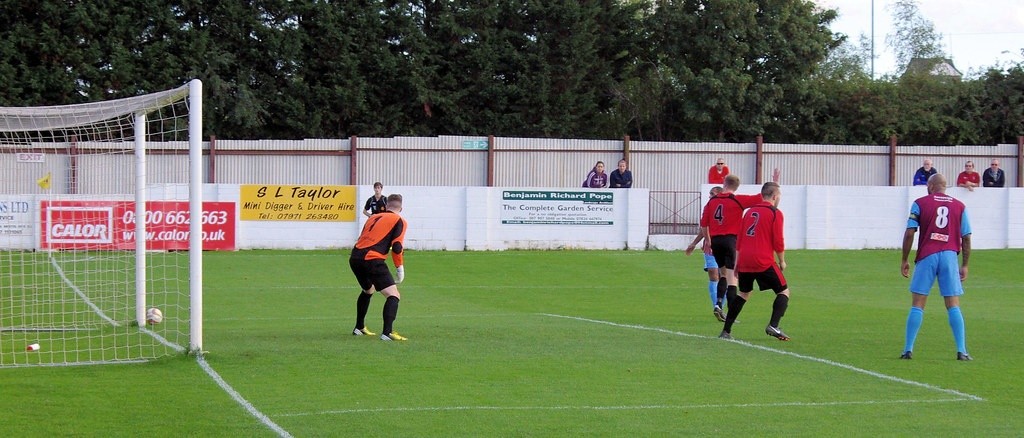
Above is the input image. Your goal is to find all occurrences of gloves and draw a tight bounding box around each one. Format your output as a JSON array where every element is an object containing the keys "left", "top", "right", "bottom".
[{"left": 394, "top": 265, "right": 404, "bottom": 284}]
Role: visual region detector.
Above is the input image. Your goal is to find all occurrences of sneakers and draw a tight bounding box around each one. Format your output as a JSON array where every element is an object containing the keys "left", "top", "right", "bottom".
[
  {"left": 718, "top": 330, "right": 733, "bottom": 341},
  {"left": 957, "top": 351, "right": 974, "bottom": 361},
  {"left": 899, "top": 350, "right": 913, "bottom": 359},
  {"left": 352, "top": 326, "right": 376, "bottom": 335},
  {"left": 765, "top": 324, "right": 791, "bottom": 341},
  {"left": 713, "top": 305, "right": 726, "bottom": 321},
  {"left": 722, "top": 316, "right": 742, "bottom": 324},
  {"left": 380, "top": 331, "right": 409, "bottom": 341}
]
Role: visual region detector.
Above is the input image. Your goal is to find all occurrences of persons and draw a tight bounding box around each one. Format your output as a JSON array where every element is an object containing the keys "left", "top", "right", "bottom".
[
  {"left": 363, "top": 182, "right": 387, "bottom": 218},
  {"left": 718, "top": 181, "right": 790, "bottom": 341},
  {"left": 700, "top": 168, "right": 781, "bottom": 323},
  {"left": 899, "top": 173, "right": 975, "bottom": 360},
  {"left": 982, "top": 159, "right": 1005, "bottom": 187},
  {"left": 913, "top": 158, "right": 937, "bottom": 186},
  {"left": 583, "top": 161, "right": 607, "bottom": 188},
  {"left": 956, "top": 160, "right": 980, "bottom": 191},
  {"left": 609, "top": 159, "right": 632, "bottom": 188},
  {"left": 686, "top": 186, "right": 740, "bottom": 323},
  {"left": 349, "top": 194, "right": 409, "bottom": 341},
  {"left": 708, "top": 158, "right": 729, "bottom": 184}
]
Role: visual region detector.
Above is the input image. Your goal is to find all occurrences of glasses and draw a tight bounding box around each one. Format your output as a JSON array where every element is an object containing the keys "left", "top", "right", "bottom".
[
  {"left": 716, "top": 162, "right": 724, "bottom": 165},
  {"left": 965, "top": 165, "right": 972, "bottom": 168},
  {"left": 991, "top": 163, "right": 998, "bottom": 166}
]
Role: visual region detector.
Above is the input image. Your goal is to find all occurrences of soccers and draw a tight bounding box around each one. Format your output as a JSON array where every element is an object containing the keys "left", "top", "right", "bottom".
[{"left": 147, "top": 307, "right": 163, "bottom": 325}]
